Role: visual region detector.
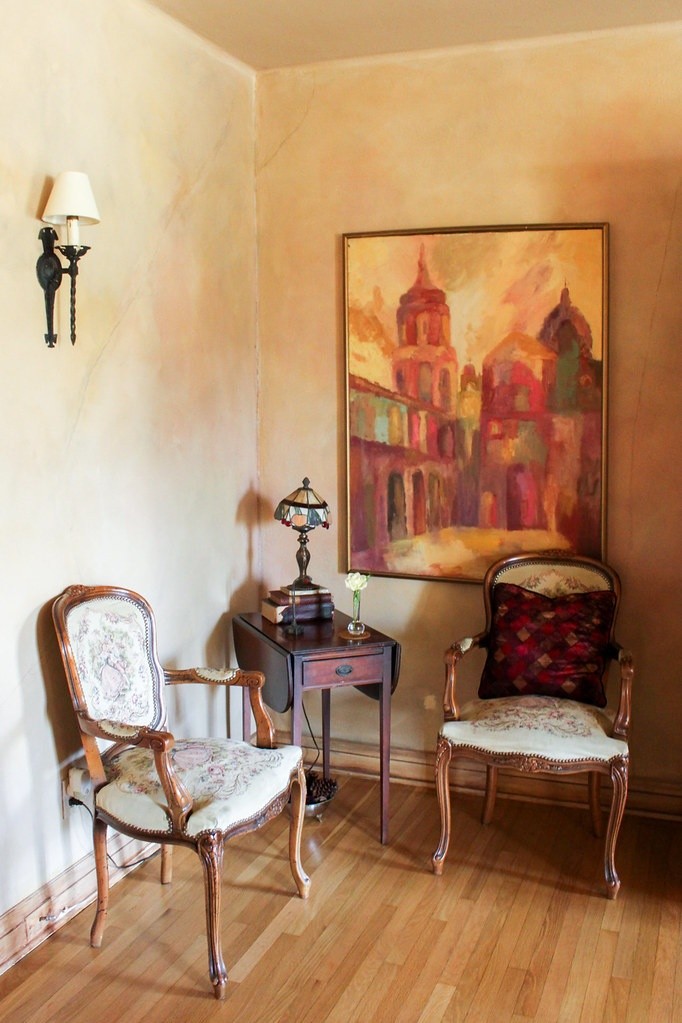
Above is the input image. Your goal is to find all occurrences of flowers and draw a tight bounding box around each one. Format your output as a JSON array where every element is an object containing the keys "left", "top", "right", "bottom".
[{"left": 344, "top": 571, "right": 369, "bottom": 628}]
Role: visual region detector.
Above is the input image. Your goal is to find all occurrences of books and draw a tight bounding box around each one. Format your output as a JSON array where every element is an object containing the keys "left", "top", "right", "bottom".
[{"left": 261, "top": 587, "right": 334, "bottom": 626}]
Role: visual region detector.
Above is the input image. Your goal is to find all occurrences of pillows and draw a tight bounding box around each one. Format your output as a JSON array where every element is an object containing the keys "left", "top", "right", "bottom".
[{"left": 477, "top": 580, "right": 617, "bottom": 704}]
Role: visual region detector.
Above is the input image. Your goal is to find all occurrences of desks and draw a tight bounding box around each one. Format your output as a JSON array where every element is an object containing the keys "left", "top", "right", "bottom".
[{"left": 230, "top": 608, "right": 401, "bottom": 842}]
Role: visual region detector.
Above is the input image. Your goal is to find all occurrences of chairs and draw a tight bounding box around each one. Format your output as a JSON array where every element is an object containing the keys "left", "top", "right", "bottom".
[
  {"left": 432, "top": 553, "right": 637, "bottom": 902},
  {"left": 51, "top": 584, "right": 311, "bottom": 1002}
]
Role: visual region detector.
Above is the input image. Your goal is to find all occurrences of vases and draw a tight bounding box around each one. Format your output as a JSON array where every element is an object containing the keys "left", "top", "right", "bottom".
[{"left": 347, "top": 590, "right": 365, "bottom": 636}]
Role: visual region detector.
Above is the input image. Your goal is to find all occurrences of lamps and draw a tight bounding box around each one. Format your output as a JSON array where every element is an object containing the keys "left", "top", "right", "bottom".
[
  {"left": 35, "top": 168, "right": 102, "bottom": 349},
  {"left": 272, "top": 476, "right": 332, "bottom": 596}
]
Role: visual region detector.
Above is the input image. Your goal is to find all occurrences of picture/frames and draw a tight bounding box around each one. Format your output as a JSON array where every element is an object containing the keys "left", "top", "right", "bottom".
[{"left": 341, "top": 223, "right": 609, "bottom": 579}]
